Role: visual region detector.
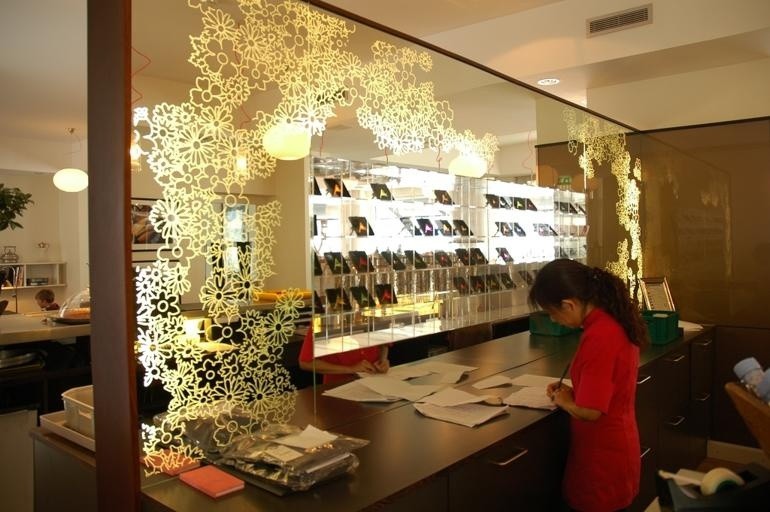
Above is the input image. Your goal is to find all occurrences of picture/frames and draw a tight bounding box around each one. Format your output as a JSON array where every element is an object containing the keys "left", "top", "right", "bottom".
[
  {"left": 130, "top": 197, "right": 182, "bottom": 251},
  {"left": 639, "top": 276, "right": 675, "bottom": 311},
  {"left": 131, "top": 260, "right": 180, "bottom": 305}
]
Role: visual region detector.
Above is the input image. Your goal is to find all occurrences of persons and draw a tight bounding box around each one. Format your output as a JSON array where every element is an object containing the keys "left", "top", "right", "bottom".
[
  {"left": 34, "top": 289, "right": 60, "bottom": 311},
  {"left": 298, "top": 318, "right": 391, "bottom": 386},
  {"left": 526, "top": 258, "right": 651, "bottom": 510}
]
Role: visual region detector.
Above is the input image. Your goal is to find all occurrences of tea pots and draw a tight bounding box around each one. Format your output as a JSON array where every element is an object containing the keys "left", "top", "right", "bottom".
[{"left": 35, "top": 241, "right": 50, "bottom": 262}]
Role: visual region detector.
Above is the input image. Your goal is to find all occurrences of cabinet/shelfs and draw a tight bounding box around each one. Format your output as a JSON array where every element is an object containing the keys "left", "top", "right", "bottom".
[
  {"left": 138, "top": 317, "right": 720, "bottom": 511},
  {"left": 0, "top": 336, "right": 91, "bottom": 417},
  {"left": 0, "top": 261, "right": 68, "bottom": 290},
  {"left": 28, "top": 425, "right": 96, "bottom": 512},
  {"left": 302, "top": 153, "right": 592, "bottom": 352}
]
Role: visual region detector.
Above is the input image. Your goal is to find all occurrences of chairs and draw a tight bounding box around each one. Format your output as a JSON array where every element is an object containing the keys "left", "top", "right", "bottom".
[{"left": 725, "top": 381, "right": 770, "bottom": 461}]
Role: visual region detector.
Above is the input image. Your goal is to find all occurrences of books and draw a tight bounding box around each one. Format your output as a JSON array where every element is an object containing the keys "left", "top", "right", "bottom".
[
  {"left": 179, "top": 464, "right": 245, "bottom": 501},
  {"left": 9, "top": 266, "right": 24, "bottom": 287},
  {"left": 140, "top": 448, "right": 199, "bottom": 477}
]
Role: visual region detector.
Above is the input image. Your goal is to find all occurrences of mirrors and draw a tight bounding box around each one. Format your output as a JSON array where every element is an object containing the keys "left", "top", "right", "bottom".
[{"left": 128, "top": 2, "right": 643, "bottom": 489}]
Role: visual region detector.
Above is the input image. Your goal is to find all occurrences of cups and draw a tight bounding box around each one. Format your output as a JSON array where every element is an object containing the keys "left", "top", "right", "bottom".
[{"left": 733, "top": 356, "right": 765, "bottom": 395}]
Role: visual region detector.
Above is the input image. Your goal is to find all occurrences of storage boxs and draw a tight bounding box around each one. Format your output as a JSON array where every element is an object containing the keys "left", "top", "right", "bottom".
[
  {"left": 638, "top": 308, "right": 679, "bottom": 346},
  {"left": 62, "top": 384, "right": 95, "bottom": 437}
]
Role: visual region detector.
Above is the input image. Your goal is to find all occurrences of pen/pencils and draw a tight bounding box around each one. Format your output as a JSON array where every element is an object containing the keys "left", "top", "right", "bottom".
[
  {"left": 551, "top": 363, "right": 570, "bottom": 401},
  {"left": 379, "top": 344, "right": 384, "bottom": 365}
]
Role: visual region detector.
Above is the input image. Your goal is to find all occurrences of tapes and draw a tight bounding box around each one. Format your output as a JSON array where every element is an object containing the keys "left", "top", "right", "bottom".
[{"left": 700, "top": 467, "right": 744, "bottom": 497}]
